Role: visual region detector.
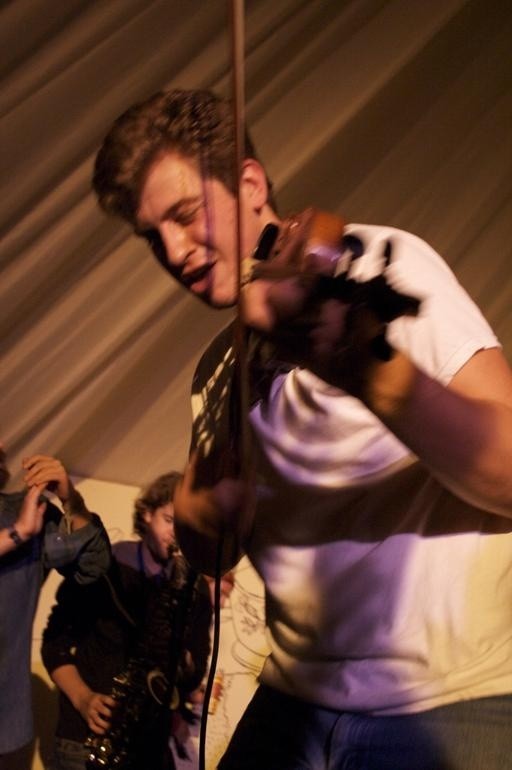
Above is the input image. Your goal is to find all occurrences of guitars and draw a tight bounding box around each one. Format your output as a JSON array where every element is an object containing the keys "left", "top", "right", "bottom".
[{"left": 172, "top": 210, "right": 349, "bottom": 581}]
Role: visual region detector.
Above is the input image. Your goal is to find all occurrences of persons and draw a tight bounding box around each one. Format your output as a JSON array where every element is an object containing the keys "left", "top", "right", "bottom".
[
  {"left": 0, "top": 447, "right": 113, "bottom": 770},
  {"left": 40, "top": 470, "right": 214, "bottom": 770},
  {"left": 167, "top": 571, "right": 237, "bottom": 769},
  {"left": 91, "top": 88, "right": 512, "bottom": 770}
]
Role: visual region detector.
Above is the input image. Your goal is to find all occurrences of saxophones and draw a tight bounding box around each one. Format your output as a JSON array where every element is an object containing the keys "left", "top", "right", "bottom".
[{"left": 84, "top": 544, "right": 183, "bottom": 769}]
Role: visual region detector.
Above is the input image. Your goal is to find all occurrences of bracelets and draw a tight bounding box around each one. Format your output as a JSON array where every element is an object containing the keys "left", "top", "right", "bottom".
[{"left": 9, "top": 524, "right": 25, "bottom": 547}]
